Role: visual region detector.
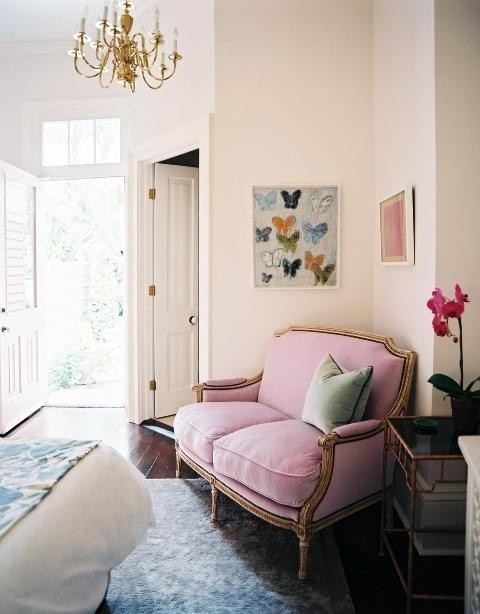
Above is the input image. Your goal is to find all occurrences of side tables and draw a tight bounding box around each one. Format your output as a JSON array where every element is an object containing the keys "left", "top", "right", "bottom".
[{"left": 379, "top": 413, "right": 468, "bottom": 612}]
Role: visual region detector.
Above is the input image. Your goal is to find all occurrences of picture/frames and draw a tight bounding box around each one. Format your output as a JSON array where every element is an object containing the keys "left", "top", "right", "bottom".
[
  {"left": 252, "top": 185, "right": 341, "bottom": 291},
  {"left": 374, "top": 184, "right": 414, "bottom": 266}
]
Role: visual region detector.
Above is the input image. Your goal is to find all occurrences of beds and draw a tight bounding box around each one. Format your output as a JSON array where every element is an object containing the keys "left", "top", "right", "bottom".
[{"left": 2, "top": 435, "right": 153, "bottom": 613}]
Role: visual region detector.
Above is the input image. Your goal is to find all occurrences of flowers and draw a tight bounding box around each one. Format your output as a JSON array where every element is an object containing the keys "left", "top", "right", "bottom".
[{"left": 426, "top": 283, "right": 480, "bottom": 400}]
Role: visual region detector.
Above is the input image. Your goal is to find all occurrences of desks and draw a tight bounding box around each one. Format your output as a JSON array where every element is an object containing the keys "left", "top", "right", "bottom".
[{"left": 458, "top": 436, "right": 480, "bottom": 614}]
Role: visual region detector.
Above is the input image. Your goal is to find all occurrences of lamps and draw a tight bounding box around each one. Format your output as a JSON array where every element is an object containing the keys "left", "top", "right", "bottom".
[{"left": 67, "top": 0, "right": 184, "bottom": 95}]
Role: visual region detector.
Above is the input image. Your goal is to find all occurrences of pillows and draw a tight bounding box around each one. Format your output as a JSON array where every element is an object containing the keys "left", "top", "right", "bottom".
[{"left": 302, "top": 350, "right": 374, "bottom": 440}]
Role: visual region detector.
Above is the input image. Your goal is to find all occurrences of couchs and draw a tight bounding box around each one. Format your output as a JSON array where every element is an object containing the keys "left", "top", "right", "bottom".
[{"left": 172, "top": 326, "right": 418, "bottom": 581}]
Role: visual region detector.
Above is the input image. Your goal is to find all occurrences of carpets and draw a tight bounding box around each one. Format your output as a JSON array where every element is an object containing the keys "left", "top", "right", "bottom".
[{"left": 107, "top": 477, "right": 357, "bottom": 614}]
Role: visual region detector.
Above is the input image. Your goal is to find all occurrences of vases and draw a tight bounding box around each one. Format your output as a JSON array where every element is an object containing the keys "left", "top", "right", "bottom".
[{"left": 451, "top": 398, "right": 479, "bottom": 435}]
